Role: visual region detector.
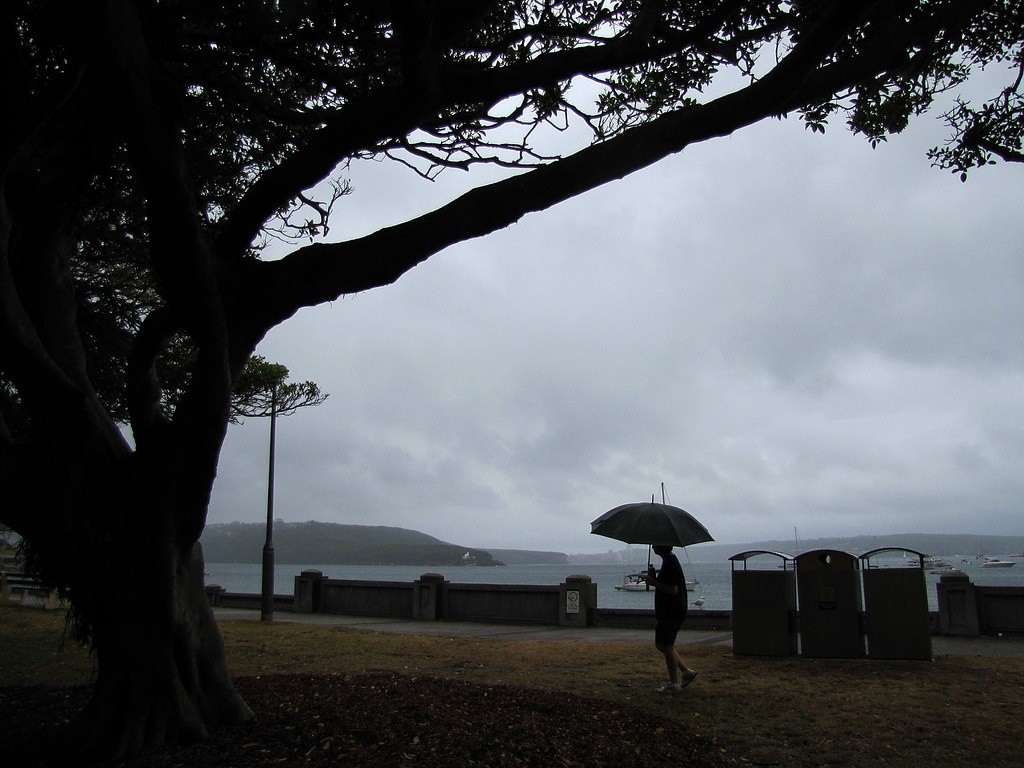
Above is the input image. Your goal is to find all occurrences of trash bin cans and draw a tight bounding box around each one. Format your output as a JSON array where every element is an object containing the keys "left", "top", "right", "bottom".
[{"left": 728, "top": 546, "right": 932, "bottom": 659}]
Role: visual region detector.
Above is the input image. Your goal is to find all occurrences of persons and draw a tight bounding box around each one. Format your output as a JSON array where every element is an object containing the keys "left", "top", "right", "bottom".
[
  {"left": 645, "top": 544, "right": 698, "bottom": 692},
  {"left": 648, "top": 564, "right": 656, "bottom": 577}
]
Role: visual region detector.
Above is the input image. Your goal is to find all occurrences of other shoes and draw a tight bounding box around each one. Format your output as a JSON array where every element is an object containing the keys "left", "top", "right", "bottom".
[
  {"left": 680, "top": 668, "right": 698, "bottom": 689},
  {"left": 656, "top": 682, "right": 681, "bottom": 695}
]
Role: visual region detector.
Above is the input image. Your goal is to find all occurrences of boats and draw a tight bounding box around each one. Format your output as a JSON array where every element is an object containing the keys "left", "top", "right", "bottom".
[
  {"left": 960, "top": 560, "right": 971, "bottom": 564},
  {"left": 928, "top": 566, "right": 961, "bottom": 574},
  {"left": 980, "top": 556, "right": 1016, "bottom": 568},
  {"left": 906, "top": 554, "right": 945, "bottom": 567}
]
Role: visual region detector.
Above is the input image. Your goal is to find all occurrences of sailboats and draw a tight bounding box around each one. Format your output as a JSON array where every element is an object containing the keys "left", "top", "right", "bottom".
[{"left": 614, "top": 481, "right": 700, "bottom": 591}]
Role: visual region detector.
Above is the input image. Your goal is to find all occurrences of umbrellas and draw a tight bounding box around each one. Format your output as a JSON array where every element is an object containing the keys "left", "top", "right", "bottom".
[{"left": 590, "top": 494, "right": 715, "bottom": 590}]
